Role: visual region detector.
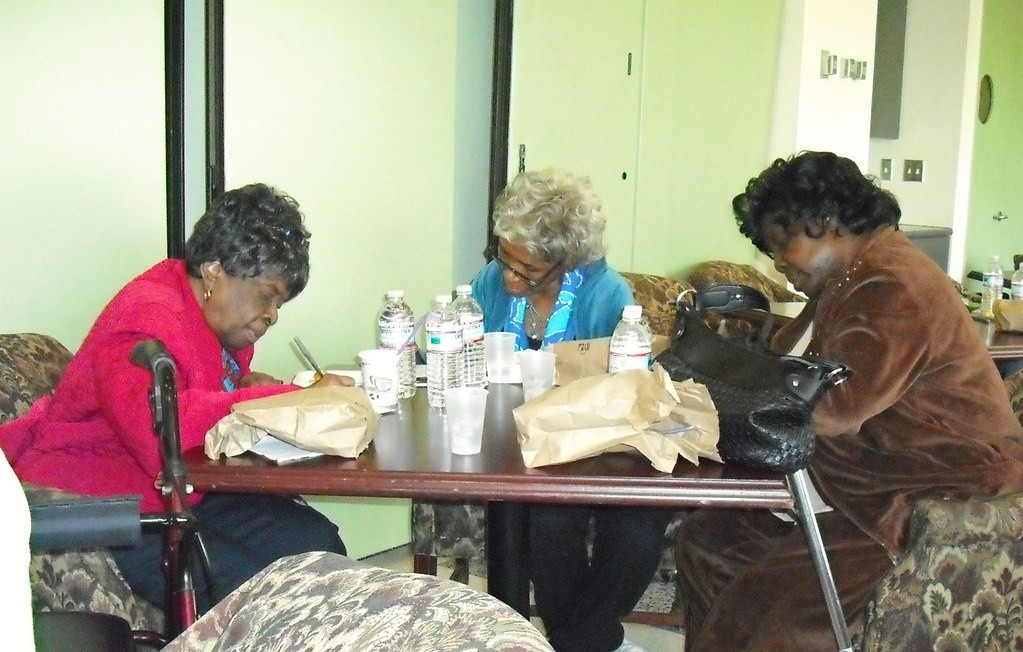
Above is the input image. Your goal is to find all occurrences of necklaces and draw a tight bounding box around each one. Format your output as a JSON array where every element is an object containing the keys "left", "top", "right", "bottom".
[{"left": 527, "top": 299, "right": 549, "bottom": 340}]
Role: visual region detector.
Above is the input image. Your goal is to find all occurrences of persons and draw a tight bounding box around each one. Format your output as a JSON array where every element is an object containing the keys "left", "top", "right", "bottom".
[
  {"left": 0, "top": 183, "right": 355, "bottom": 615},
  {"left": 415, "top": 172, "right": 675, "bottom": 652},
  {"left": 675, "top": 152, "right": 1023, "bottom": 652}
]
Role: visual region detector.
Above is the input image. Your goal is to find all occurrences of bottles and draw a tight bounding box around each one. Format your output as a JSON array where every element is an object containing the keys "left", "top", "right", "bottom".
[
  {"left": 426, "top": 296, "right": 455, "bottom": 408},
  {"left": 1010, "top": 263, "right": 1023, "bottom": 301},
  {"left": 983, "top": 256, "right": 1004, "bottom": 319},
  {"left": 607, "top": 305, "right": 652, "bottom": 372},
  {"left": 377, "top": 291, "right": 418, "bottom": 398},
  {"left": 451, "top": 286, "right": 487, "bottom": 389}
]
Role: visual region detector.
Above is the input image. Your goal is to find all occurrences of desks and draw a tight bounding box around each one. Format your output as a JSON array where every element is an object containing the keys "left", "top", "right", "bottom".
[{"left": 158, "top": 363, "right": 853, "bottom": 652}]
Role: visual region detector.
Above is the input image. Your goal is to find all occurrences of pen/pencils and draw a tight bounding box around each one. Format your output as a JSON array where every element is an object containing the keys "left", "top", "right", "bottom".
[{"left": 294, "top": 336, "right": 324, "bottom": 378}]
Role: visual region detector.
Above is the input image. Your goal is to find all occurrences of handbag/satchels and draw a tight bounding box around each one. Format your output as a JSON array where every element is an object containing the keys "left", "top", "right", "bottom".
[{"left": 648, "top": 281, "right": 855, "bottom": 477}]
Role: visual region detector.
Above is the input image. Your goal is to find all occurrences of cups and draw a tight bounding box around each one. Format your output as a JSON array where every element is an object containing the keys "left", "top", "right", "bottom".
[
  {"left": 444, "top": 387, "right": 489, "bottom": 456},
  {"left": 519, "top": 353, "right": 557, "bottom": 404},
  {"left": 358, "top": 349, "right": 402, "bottom": 414},
  {"left": 483, "top": 332, "right": 517, "bottom": 384}
]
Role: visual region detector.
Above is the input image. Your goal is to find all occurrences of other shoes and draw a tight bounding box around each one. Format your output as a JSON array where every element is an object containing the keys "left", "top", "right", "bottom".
[{"left": 612, "top": 641, "right": 646, "bottom": 652}]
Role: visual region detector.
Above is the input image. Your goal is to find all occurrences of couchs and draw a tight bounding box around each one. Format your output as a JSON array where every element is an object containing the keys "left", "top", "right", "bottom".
[{"left": 0, "top": 262, "right": 1023, "bottom": 652}]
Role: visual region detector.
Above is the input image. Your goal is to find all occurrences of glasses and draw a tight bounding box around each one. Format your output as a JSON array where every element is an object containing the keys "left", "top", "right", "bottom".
[{"left": 483, "top": 235, "right": 568, "bottom": 288}]
